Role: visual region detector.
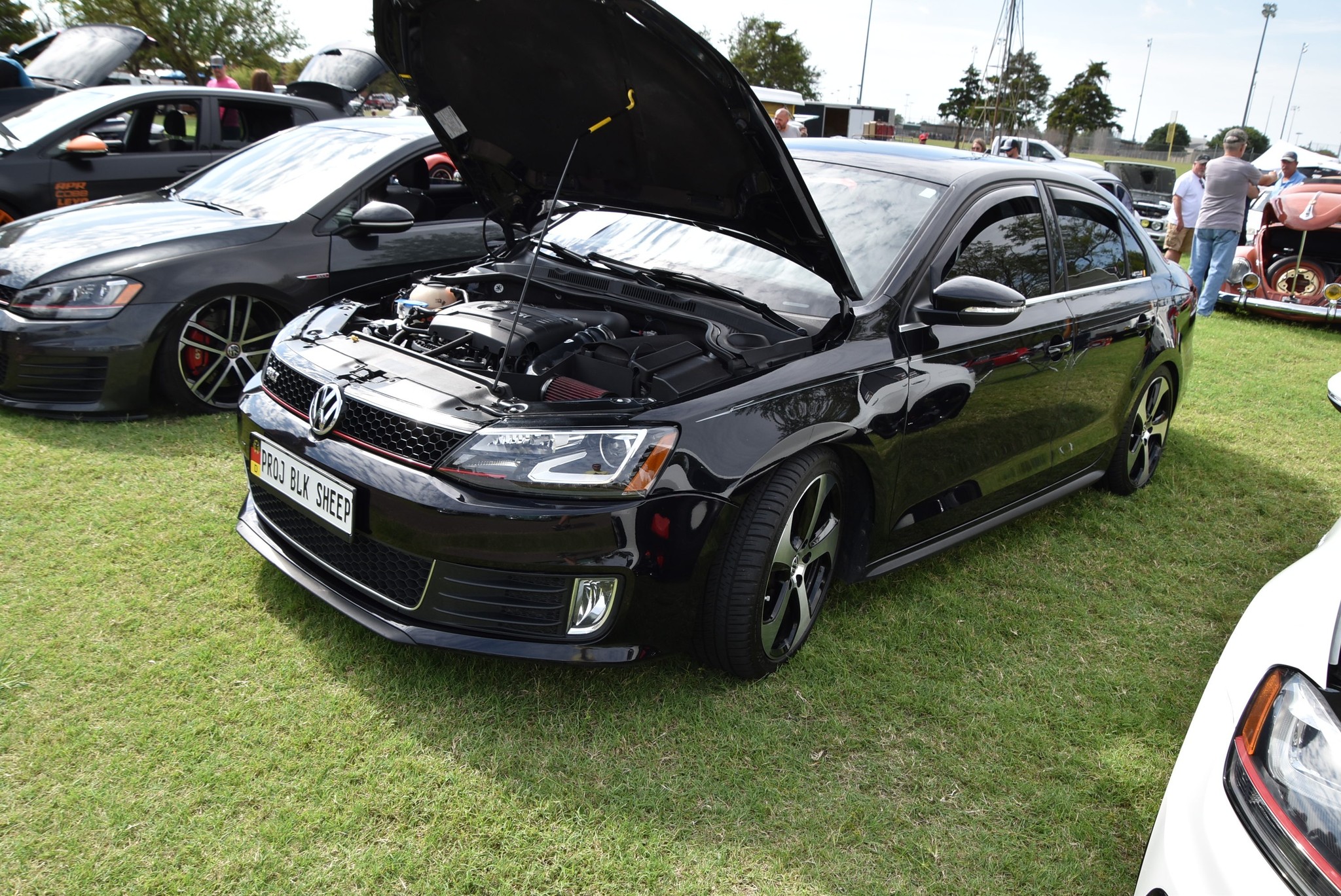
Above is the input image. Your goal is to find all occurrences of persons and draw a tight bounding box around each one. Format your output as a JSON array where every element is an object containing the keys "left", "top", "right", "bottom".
[
  {"left": 1163, "top": 154, "right": 1211, "bottom": 264},
  {"left": 772, "top": 107, "right": 802, "bottom": 138},
  {"left": 1188, "top": 128, "right": 1277, "bottom": 319},
  {"left": 1270, "top": 151, "right": 1308, "bottom": 197},
  {"left": 1204, "top": 180, "right": 1260, "bottom": 283},
  {"left": 918, "top": 129, "right": 929, "bottom": 145},
  {"left": 248, "top": 68, "right": 276, "bottom": 93},
  {"left": 970, "top": 137, "right": 987, "bottom": 154},
  {"left": 206, "top": 55, "right": 243, "bottom": 141},
  {"left": 999, "top": 138, "right": 1023, "bottom": 160}
]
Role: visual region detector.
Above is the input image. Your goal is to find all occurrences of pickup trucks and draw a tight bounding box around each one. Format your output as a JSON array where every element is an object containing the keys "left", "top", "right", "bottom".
[{"left": 989, "top": 134, "right": 1065, "bottom": 165}]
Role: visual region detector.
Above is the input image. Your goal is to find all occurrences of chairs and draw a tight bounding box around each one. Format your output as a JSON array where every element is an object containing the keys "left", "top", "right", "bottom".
[
  {"left": 394, "top": 156, "right": 439, "bottom": 220},
  {"left": 218, "top": 118, "right": 231, "bottom": 142},
  {"left": 151, "top": 111, "right": 185, "bottom": 152}
]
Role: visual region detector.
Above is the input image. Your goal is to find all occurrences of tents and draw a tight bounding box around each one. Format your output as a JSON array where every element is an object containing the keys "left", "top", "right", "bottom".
[{"left": 1250, "top": 140, "right": 1341, "bottom": 176}]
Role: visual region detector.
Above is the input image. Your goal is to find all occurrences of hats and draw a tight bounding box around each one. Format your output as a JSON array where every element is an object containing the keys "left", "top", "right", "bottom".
[
  {"left": 1000, "top": 138, "right": 1019, "bottom": 150},
  {"left": 1223, "top": 128, "right": 1250, "bottom": 145},
  {"left": 1280, "top": 151, "right": 1298, "bottom": 162},
  {"left": 1195, "top": 154, "right": 1210, "bottom": 164},
  {"left": 919, "top": 134, "right": 927, "bottom": 140},
  {"left": 208, "top": 55, "right": 225, "bottom": 66}
]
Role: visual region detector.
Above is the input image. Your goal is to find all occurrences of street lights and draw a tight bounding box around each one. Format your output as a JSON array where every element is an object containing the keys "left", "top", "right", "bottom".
[
  {"left": 1287, "top": 106, "right": 1301, "bottom": 143},
  {"left": 1241, "top": 3, "right": 1278, "bottom": 127},
  {"left": 1280, "top": 41, "right": 1308, "bottom": 138},
  {"left": 1132, "top": 38, "right": 1152, "bottom": 141}
]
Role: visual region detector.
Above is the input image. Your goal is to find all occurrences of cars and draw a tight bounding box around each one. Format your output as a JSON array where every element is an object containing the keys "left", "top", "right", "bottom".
[
  {"left": 1059, "top": 161, "right": 1341, "bottom": 325},
  {"left": 0, "top": 28, "right": 560, "bottom": 411},
  {"left": 233, "top": 0, "right": 1196, "bottom": 681},
  {"left": 1136, "top": 375, "right": 1341, "bottom": 895}
]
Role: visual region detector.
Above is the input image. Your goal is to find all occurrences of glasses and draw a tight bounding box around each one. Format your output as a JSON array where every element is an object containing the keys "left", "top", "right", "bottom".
[
  {"left": 1199, "top": 179, "right": 1205, "bottom": 189},
  {"left": 1004, "top": 147, "right": 1014, "bottom": 152},
  {"left": 971, "top": 147, "right": 980, "bottom": 150},
  {"left": 921, "top": 139, "right": 926, "bottom": 141},
  {"left": 211, "top": 66, "right": 223, "bottom": 69}
]
